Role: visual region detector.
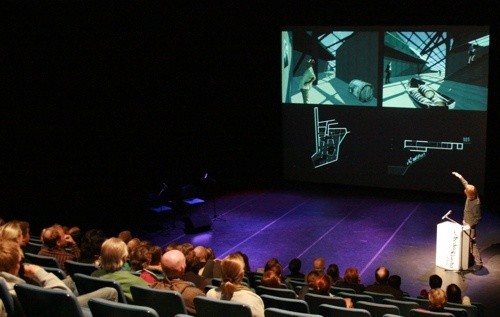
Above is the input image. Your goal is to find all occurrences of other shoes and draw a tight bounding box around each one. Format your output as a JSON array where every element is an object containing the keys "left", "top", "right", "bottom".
[{"left": 472, "top": 264, "right": 482, "bottom": 271}]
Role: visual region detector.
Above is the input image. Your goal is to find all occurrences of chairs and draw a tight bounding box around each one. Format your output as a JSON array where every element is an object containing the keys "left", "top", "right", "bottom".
[
  {"left": 175, "top": 180, "right": 207, "bottom": 230},
  {"left": 0, "top": 225, "right": 490, "bottom": 317},
  {"left": 147, "top": 186, "right": 177, "bottom": 231}
]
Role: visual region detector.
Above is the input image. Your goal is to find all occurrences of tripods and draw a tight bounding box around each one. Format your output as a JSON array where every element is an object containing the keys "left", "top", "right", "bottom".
[{"left": 210, "top": 182, "right": 226, "bottom": 222}]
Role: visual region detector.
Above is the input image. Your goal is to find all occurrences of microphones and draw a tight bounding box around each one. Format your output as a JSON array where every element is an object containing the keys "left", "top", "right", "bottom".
[{"left": 441, "top": 210, "right": 452, "bottom": 220}]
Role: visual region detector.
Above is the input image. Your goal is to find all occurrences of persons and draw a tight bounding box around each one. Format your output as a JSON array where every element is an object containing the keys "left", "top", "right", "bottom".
[
  {"left": 312, "top": 57, "right": 318, "bottom": 85},
  {"left": 452, "top": 172, "right": 483, "bottom": 271},
  {"left": 385, "top": 61, "right": 393, "bottom": 84},
  {"left": 0, "top": 219, "right": 471, "bottom": 317},
  {"left": 298, "top": 59, "right": 316, "bottom": 104},
  {"left": 439, "top": 70, "right": 441, "bottom": 77}
]
